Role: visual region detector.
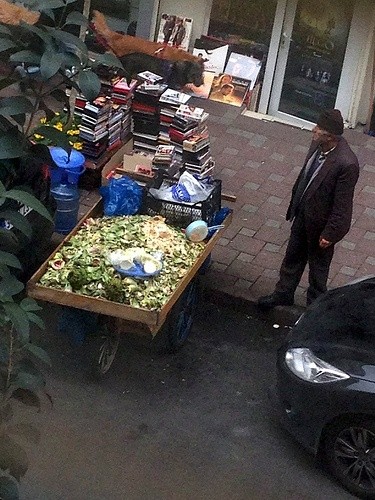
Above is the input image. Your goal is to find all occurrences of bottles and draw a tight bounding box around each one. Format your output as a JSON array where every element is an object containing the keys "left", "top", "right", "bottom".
[{"left": 50, "top": 177, "right": 80, "bottom": 235}]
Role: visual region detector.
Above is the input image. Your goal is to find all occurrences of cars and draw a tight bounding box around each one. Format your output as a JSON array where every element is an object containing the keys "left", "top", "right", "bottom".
[{"left": 275, "top": 277, "right": 375, "bottom": 500}]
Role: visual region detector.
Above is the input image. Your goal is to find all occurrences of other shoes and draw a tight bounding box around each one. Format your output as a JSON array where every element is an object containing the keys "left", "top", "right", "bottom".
[{"left": 257, "top": 293, "right": 294, "bottom": 309}]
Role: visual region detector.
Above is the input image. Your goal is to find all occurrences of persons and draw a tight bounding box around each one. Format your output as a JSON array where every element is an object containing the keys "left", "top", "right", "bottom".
[
  {"left": 258, "top": 109, "right": 360, "bottom": 308},
  {"left": 172, "top": 20, "right": 185, "bottom": 49},
  {"left": 163, "top": 15, "right": 176, "bottom": 44},
  {"left": 211, "top": 74, "right": 234, "bottom": 102}
]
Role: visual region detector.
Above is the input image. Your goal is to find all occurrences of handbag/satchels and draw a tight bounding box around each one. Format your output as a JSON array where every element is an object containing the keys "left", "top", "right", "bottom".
[{"left": 97, "top": 175, "right": 144, "bottom": 216}]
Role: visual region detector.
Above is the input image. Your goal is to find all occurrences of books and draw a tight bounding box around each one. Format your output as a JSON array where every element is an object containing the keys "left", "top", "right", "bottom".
[{"left": 64, "top": 70, "right": 214, "bottom": 174}]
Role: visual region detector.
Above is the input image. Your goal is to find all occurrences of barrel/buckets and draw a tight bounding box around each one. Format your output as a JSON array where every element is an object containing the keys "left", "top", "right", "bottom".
[{"left": 49, "top": 147, "right": 86, "bottom": 186}]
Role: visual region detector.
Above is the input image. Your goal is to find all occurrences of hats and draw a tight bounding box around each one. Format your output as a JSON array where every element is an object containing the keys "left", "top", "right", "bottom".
[{"left": 316, "top": 108, "right": 344, "bottom": 135}]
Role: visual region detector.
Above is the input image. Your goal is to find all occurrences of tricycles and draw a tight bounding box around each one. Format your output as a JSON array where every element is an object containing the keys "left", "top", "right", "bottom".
[{"left": 91, "top": 170, "right": 237, "bottom": 377}]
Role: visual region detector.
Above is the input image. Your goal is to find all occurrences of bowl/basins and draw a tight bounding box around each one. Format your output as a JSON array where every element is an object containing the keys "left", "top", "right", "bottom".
[{"left": 113, "top": 259, "right": 162, "bottom": 277}]
